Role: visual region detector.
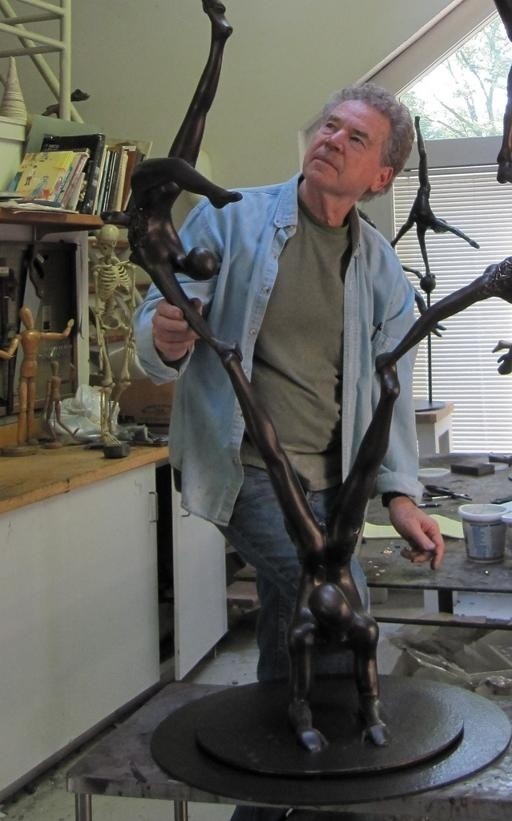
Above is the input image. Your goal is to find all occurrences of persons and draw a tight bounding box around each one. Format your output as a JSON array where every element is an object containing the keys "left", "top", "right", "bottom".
[{"left": 133, "top": 81, "right": 445, "bottom": 681}]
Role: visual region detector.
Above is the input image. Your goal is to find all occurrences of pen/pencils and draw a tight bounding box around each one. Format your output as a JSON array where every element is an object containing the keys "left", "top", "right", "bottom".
[{"left": 417, "top": 485, "right": 472, "bottom": 508}]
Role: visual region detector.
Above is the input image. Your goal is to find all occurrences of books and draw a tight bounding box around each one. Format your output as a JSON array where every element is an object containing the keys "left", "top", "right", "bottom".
[{"left": 0, "top": 133, "right": 153, "bottom": 216}]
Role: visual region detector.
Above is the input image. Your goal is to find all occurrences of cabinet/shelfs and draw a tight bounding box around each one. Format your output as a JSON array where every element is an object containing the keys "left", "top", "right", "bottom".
[{"left": 0, "top": 442, "right": 232, "bottom": 810}]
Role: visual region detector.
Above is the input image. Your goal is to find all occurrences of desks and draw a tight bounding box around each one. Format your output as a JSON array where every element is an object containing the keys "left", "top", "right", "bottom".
[{"left": 66, "top": 680, "right": 511, "bottom": 820}]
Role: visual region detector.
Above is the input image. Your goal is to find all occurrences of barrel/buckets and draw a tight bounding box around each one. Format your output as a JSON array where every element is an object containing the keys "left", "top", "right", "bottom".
[
  {"left": 501, "top": 510, "right": 512, "bottom": 568},
  {"left": 456, "top": 501, "right": 506, "bottom": 564}
]
